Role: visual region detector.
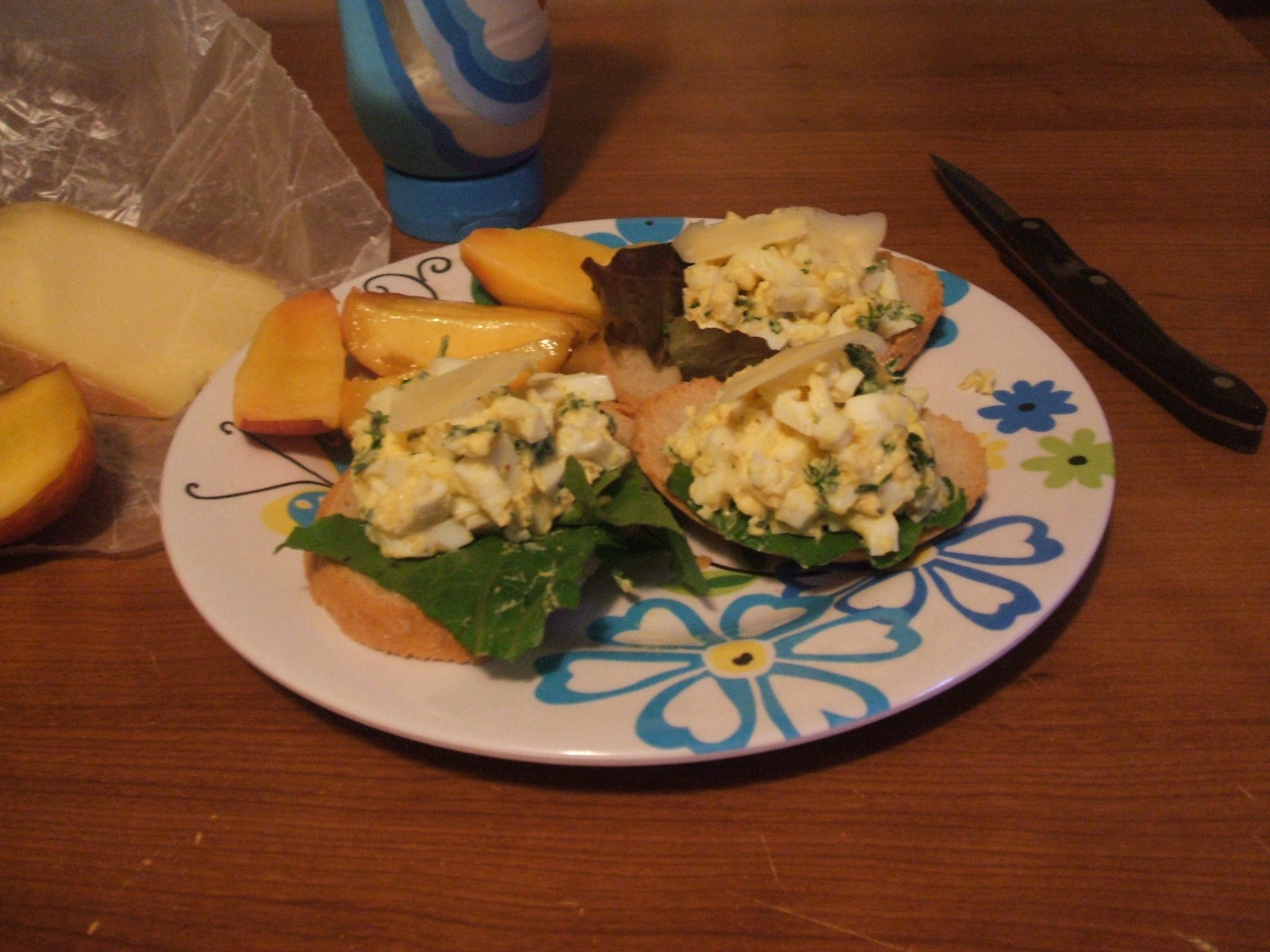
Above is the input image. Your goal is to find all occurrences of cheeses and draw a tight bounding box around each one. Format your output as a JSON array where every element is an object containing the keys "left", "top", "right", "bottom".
[{"left": 0, "top": 196, "right": 283, "bottom": 541}]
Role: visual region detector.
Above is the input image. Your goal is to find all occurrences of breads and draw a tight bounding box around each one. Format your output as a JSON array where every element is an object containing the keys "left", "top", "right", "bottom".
[
  {"left": 565, "top": 252, "right": 986, "bottom": 557},
  {"left": 304, "top": 458, "right": 483, "bottom": 661}
]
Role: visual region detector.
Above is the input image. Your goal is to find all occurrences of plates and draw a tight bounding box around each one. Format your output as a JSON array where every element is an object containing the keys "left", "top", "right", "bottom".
[{"left": 158, "top": 215, "right": 1117, "bottom": 766}]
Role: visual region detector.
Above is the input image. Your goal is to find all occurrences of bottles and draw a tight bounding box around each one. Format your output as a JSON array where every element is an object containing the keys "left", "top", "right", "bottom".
[{"left": 338, "top": 1, "right": 552, "bottom": 240}]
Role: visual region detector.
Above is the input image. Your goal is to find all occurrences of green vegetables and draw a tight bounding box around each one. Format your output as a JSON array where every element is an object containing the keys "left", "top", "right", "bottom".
[{"left": 274, "top": 454, "right": 968, "bottom": 662}]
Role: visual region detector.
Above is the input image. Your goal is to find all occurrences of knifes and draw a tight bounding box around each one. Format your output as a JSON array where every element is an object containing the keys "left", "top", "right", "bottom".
[{"left": 927, "top": 151, "right": 1267, "bottom": 444}]
